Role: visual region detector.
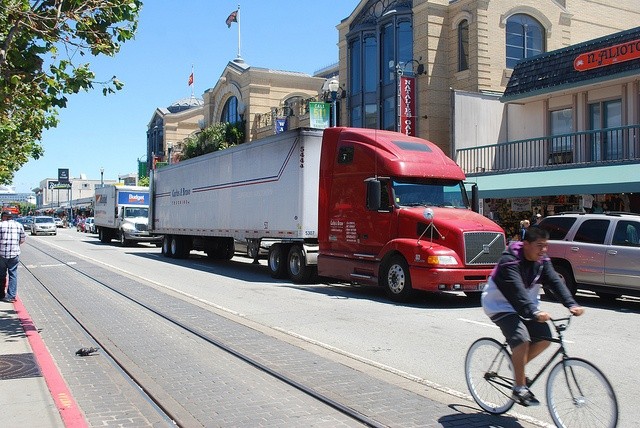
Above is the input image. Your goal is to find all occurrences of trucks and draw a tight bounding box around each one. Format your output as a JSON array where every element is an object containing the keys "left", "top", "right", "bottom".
[
  {"left": 94, "top": 184, "right": 164, "bottom": 248},
  {"left": 148, "top": 127, "right": 506, "bottom": 302}
]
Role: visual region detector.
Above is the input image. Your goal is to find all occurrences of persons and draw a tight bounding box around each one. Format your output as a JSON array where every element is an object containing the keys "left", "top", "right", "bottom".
[
  {"left": 520, "top": 221, "right": 524, "bottom": 228},
  {"left": 0, "top": 210, "right": 26, "bottom": 302},
  {"left": 481, "top": 224, "right": 585, "bottom": 406},
  {"left": 521, "top": 220, "right": 530, "bottom": 240},
  {"left": 0, "top": 212, "right": 6, "bottom": 222}
]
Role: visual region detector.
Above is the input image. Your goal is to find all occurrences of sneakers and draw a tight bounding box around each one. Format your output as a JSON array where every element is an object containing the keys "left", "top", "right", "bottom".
[
  {"left": 525, "top": 376, "right": 531, "bottom": 386},
  {"left": 511, "top": 388, "right": 540, "bottom": 405}
]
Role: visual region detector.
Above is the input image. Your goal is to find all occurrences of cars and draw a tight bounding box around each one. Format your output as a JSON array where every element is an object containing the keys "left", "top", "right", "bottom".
[
  {"left": 12, "top": 213, "right": 22, "bottom": 223},
  {"left": 85, "top": 215, "right": 98, "bottom": 233},
  {"left": 53, "top": 217, "right": 64, "bottom": 227},
  {"left": 31, "top": 215, "right": 57, "bottom": 235},
  {"left": 76, "top": 219, "right": 87, "bottom": 232},
  {"left": 22, "top": 215, "right": 32, "bottom": 229}
]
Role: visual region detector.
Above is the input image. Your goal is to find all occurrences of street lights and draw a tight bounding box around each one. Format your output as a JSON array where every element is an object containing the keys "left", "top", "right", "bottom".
[
  {"left": 327, "top": 75, "right": 342, "bottom": 127},
  {"left": 99, "top": 166, "right": 105, "bottom": 185},
  {"left": 166, "top": 140, "right": 174, "bottom": 166}
]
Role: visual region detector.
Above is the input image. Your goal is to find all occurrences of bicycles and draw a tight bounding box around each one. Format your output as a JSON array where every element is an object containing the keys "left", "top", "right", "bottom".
[{"left": 462, "top": 312, "right": 619, "bottom": 427}]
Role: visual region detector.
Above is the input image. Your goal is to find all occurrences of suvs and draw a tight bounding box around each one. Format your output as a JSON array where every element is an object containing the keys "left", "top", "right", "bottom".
[{"left": 534, "top": 209, "right": 640, "bottom": 302}]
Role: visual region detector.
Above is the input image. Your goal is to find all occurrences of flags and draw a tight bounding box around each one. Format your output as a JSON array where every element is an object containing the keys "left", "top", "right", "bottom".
[
  {"left": 188, "top": 73, "right": 193, "bottom": 85},
  {"left": 225, "top": 10, "right": 238, "bottom": 28}
]
